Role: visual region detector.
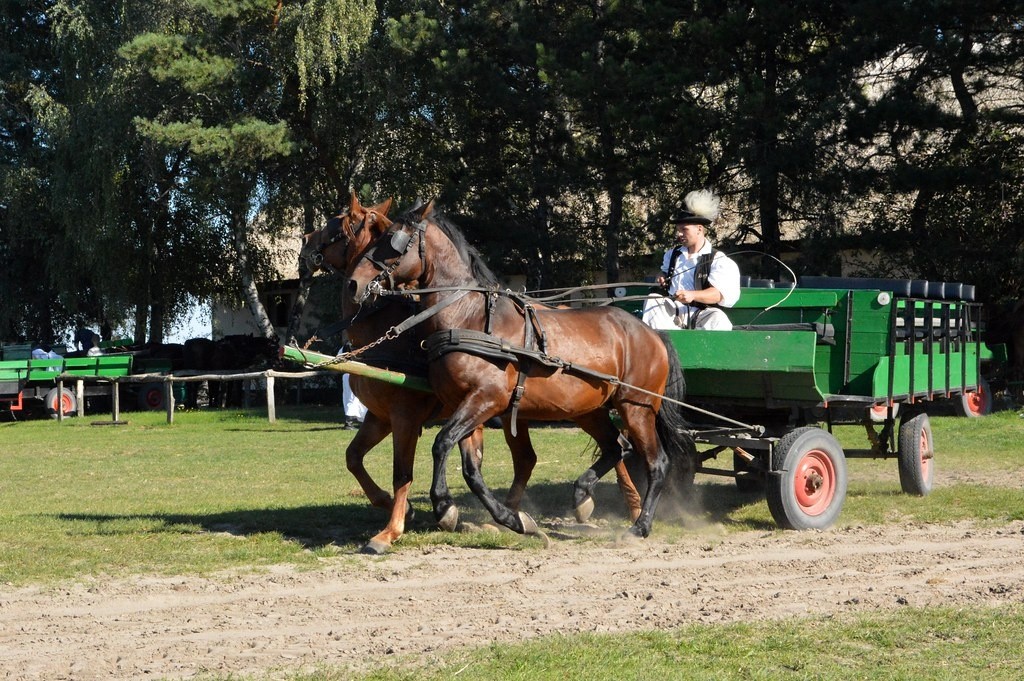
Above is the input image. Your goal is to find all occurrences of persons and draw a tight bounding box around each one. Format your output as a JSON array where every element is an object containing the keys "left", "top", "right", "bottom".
[
  {"left": 31, "top": 321, "right": 109, "bottom": 372},
  {"left": 642, "top": 188, "right": 740, "bottom": 331},
  {"left": 337, "top": 346, "right": 368, "bottom": 430}
]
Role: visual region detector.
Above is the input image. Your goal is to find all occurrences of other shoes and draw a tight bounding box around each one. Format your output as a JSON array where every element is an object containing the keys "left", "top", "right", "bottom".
[{"left": 345, "top": 425, "right": 358, "bottom": 430}]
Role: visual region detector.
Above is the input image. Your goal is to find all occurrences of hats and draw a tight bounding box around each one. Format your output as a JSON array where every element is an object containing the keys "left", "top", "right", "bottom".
[{"left": 669, "top": 189, "right": 720, "bottom": 225}]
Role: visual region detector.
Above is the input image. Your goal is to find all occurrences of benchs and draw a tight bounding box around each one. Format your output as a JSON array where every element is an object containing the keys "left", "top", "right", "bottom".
[
  {"left": 740, "top": 276, "right": 986, "bottom": 355},
  {"left": 0, "top": 338, "right": 134, "bottom": 394},
  {"left": 615, "top": 287, "right": 837, "bottom": 348}
]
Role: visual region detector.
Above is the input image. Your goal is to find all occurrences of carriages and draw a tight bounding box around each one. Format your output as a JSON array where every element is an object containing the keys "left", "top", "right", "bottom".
[{"left": 278, "top": 182, "right": 993, "bottom": 555}]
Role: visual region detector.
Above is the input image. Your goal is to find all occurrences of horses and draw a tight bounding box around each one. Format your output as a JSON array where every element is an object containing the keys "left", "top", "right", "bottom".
[{"left": 295, "top": 190, "right": 697, "bottom": 556}]
